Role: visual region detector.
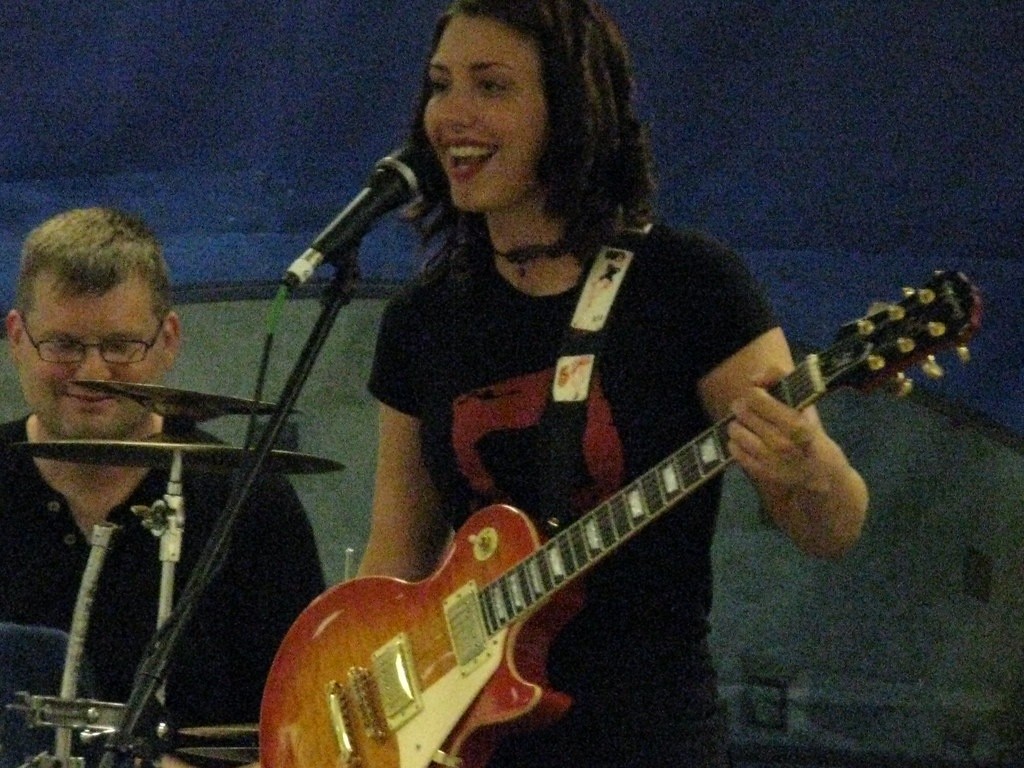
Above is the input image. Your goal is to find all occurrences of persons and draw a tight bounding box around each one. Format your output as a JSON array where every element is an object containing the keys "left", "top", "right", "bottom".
[
  {"left": 0, "top": 209, "right": 327, "bottom": 767},
  {"left": 358, "top": 0, "right": 869, "bottom": 768}
]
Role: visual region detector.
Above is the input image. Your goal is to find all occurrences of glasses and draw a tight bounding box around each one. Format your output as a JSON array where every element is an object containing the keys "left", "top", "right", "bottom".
[{"left": 15, "top": 307, "right": 170, "bottom": 365}]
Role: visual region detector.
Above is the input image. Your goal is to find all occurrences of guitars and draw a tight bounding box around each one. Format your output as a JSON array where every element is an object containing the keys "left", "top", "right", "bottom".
[{"left": 259, "top": 267, "right": 982, "bottom": 768}]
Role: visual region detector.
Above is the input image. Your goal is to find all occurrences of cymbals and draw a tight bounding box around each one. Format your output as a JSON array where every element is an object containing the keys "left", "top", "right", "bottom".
[
  {"left": 67, "top": 376, "right": 305, "bottom": 420},
  {"left": 11, "top": 438, "right": 348, "bottom": 475}
]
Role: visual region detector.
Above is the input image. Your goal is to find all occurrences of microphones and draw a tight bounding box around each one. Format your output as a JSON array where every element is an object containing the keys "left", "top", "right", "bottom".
[{"left": 282, "top": 146, "right": 434, "bottom": 290}]
